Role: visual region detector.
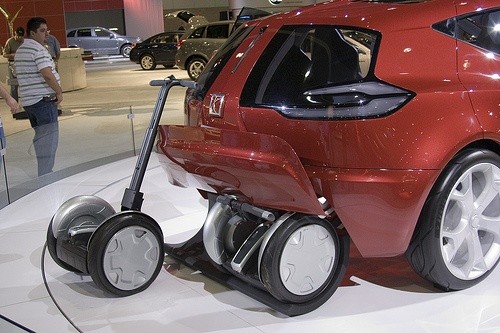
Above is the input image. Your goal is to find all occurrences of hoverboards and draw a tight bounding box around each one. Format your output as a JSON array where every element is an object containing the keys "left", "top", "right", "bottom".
[{"left": 45, "top": 75, "right": 199, "bottom": 297}]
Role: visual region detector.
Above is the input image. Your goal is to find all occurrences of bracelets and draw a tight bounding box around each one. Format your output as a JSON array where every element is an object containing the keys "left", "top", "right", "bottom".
[{"left": 56, "top": 92, "right": 63, "bottom": 98}]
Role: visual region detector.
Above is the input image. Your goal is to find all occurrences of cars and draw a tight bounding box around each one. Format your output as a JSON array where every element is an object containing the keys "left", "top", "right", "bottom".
[
  {"left": 129, "top": 31, "right": 187, "bottom": 71},
  {"left": 166, "top": 10, "right": 247, "bottom": 81},
  {"left": 66, "top": 26, "right": 144, "bottom": 59},
  {"left": 154, "top": 0, "right": 500, "bottom": 292}
]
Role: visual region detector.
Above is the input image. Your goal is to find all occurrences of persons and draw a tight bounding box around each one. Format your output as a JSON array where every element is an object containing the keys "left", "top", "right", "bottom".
[
  {"left": 43, "top": 27, "right": 61, "bottom": 73},
  {"left": 14, "top": 17, "right": 64, "bottom": 177},
  {"left": 0, "top": 81, "right": 20, "bottom": 163},
  {"left": 2, "top": 27, "right": 25, "bottom": 102}
]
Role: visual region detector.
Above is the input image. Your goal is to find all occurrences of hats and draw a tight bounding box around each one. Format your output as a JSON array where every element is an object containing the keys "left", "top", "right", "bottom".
[{"left": 16, "top": 27, "right": 25, "bottom": 36}]
